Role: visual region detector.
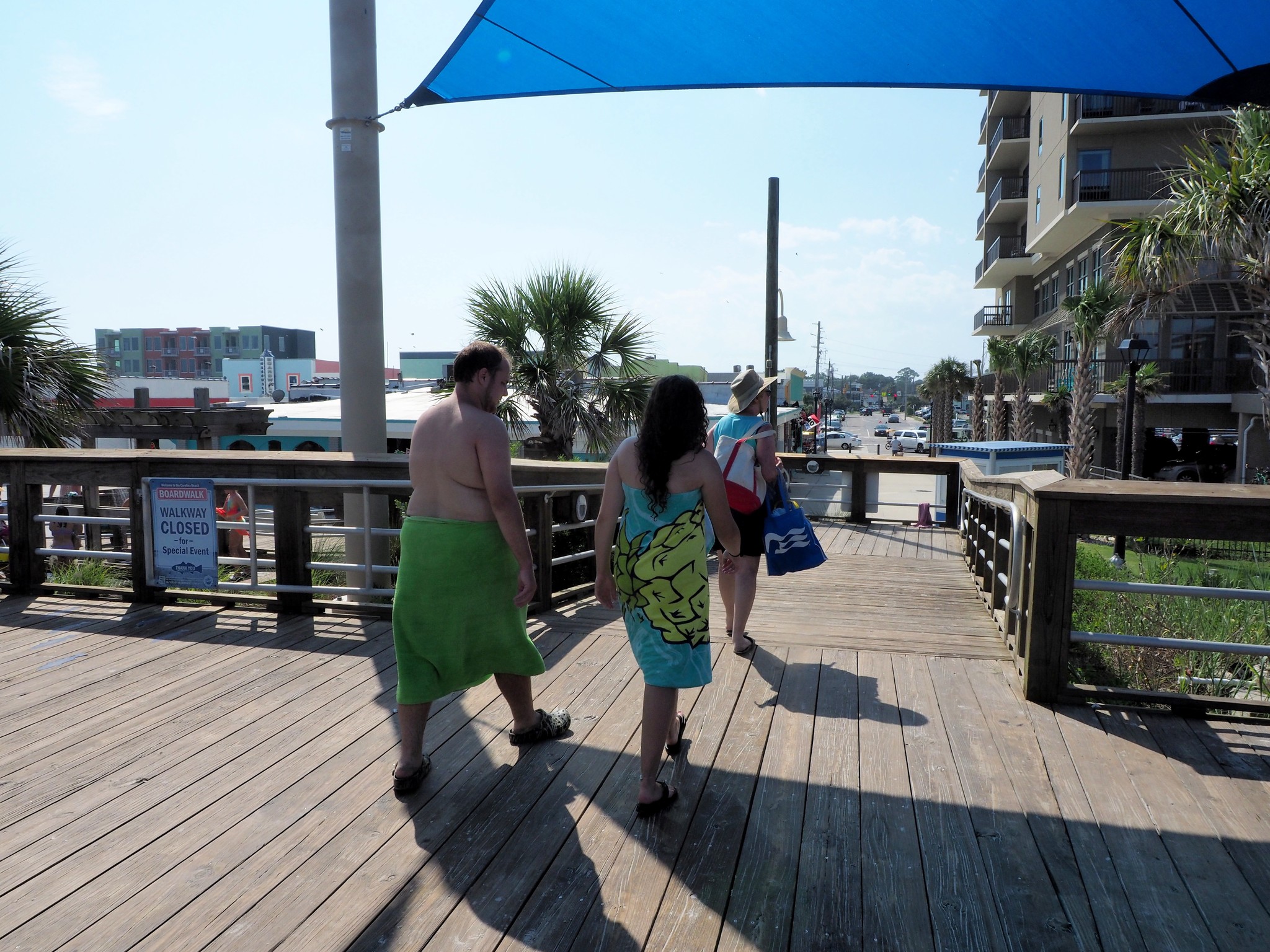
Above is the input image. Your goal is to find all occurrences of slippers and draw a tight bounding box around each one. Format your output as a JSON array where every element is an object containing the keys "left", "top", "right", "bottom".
[
  {"left": 664, "top": 711, "right": 686, "bottom": 755},
  {"left": 726, "top": 628, "right": 756, "bottom": 655},
  {"left": 226, "top": 575, "right": 244, "bottom": 582},
  {"left": 241, "top": 571, "right": 251, "bottom": 578},
  {"left": 508, "top": 708, "right": 570, "bottom": 744},
  {"left": 392, "top": 751, "right": 432, "bottom": 790},
  {"left": 636, "top": 780, "right": 678, "bottom": 814}
]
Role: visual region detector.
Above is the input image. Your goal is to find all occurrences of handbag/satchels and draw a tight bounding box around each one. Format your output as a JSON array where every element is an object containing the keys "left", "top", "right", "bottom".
[
  {"left": 713, "top": 419, "right": 777, "bottom": 514},
  {"left": 762, "top": 466, "right": 828, "bottom": 577}
]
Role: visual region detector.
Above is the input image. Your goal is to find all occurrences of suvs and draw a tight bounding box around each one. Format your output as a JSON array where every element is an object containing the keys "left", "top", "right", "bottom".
[{"left": 882, "top": 407, "right": 893, "bottom": 417}]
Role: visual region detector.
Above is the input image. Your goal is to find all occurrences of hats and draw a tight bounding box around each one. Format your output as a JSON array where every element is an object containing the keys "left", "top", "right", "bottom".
[
  {"left": 727, "top": 368, "right": 778, "bottom": 414},
  {"left": 0, "top": 487, "right": 4, "bottom": 491},
  {"left": 0, "top": 501, "right": 8, "bottom": 506}
]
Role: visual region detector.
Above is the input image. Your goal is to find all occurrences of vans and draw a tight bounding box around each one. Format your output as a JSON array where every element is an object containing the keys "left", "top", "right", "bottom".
[{"left": 892, "top": 429, "right": 927, "bottom": 454}]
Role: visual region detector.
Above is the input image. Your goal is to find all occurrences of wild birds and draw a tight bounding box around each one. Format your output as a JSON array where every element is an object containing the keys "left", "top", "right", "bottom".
[
  {"left": 413, "top": 346, "right": 416, "bottom": 348},
  {"left": 320, "top": 328, "right": 323, "bottom": 332},
  {"left": 411, "top": 332, "right": 415, "bottom": 335},
  {"left": 398, "top": 347, "right": 402, "bottom": 349}
]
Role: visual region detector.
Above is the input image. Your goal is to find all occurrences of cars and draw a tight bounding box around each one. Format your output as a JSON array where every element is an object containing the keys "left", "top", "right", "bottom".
[
  {"left": 806, "top": 409, "right": 862, "bottom": 450},
  {"left": 900, "top": 405, "right": 905, "bottom": 412},
  {"left": 874, "top": 424, "right": 890, "bottom": 437},
  {"left": 914, "top": 405, "right": 974, "bottom": 454},
  {"left": 888, "top": 414, "right": 899, "bottom": 423},
  {"left": 859, "top": 408, "right": 872, "bottom": 416},
  {"left": 857, "top": 405, "right": 888, "bottom": 412},
  {"left": 1145, "top": 426, "right": 1237, "bottom": 483}
]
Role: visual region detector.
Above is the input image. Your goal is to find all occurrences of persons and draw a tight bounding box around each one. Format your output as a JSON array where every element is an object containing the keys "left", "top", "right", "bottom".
[
  {"left": 890, "top": 436, "right": 900, "bottom": 457},
  {"left": 387, "top": 338, "right": 570, "bottom": 791},
  {"left": 0, "top": 500, "right": 9, "bottom": 543},
  {"left": 706, "top": 367, "right": 785, "bottom": 657},
  {"left": 594, "top": 373, "right": 742, "bottom": 821},
  {"left": 49, "top": 506, "right": 75, "bottom": 550},
  {"left": 215, "top": 488, "right": 248, "bottom": 580},
  {"left": 898, "top": 441, "right": 903, "bottom": 452}
]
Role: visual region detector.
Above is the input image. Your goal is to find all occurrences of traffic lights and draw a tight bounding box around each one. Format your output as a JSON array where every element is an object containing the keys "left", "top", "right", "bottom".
[
  {"left": 894, "top": 394, "right": 896, "bottom": 401},
  {"left": 874, "top": 390, "right": 875, "bottom": 394},
  {"left": 888, "top": 393, "right": 890, "bottom": 395}
]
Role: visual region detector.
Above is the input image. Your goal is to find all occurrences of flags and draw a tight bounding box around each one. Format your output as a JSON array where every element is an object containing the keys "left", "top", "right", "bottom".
[{"left": 804, "top": 414, "right": 821, "bottom": 430}]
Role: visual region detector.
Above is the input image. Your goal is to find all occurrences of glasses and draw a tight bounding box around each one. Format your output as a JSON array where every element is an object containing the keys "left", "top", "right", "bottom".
[
  {"left": 765, "top": 390, "right": 772, "bottom": 396},
  {"left": 0, "top": 506, "right": 5, "bottom": 509}
]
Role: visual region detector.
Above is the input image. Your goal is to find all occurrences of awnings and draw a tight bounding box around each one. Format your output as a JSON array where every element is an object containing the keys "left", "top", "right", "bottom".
[{"left": 383, "top": 0, "right": 1270, "bottom": 117}]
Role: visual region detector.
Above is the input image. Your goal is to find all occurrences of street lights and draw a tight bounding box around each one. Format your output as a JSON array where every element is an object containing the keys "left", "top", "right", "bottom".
[
  {"left": 1110, "top": 333, "right": 1153, "bottom": 570},
  {"left": 823, "top": 397, "right": 830, "bottom": 453},
  {"left": 829, "top": 397, "right": 833, "bottom": 432},
  {"left": 928, "top": 396, "right": 934, "bottom": 457},
  {"left": 812, "top": 390, "right": 820, "bottom": 454}
]
